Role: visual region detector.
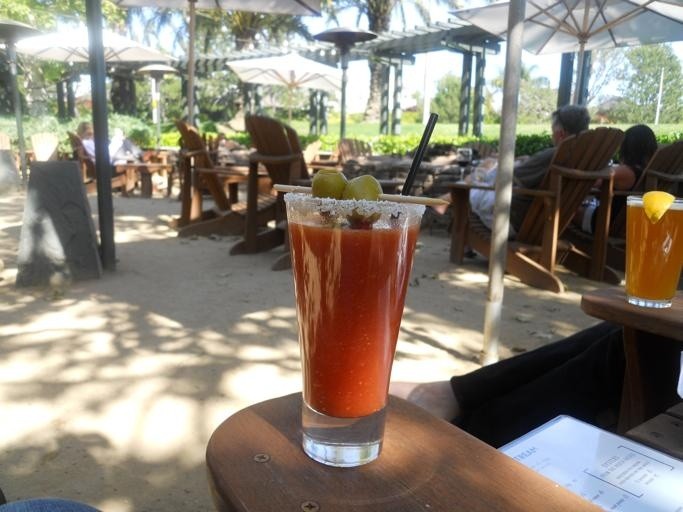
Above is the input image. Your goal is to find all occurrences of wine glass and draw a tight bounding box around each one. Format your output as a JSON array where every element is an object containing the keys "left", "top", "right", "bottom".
[{"left": 455, "top": 149, "right": 473, "bottom": 185}]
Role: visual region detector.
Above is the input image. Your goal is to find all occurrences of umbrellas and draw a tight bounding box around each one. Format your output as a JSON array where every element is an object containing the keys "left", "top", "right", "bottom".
[
  {"left": 449, "top": 0, "right": 683, "bottom": 104},
  {"left": 226, "top": 53, "right": 340, "bottom": 118},
  {"left": 2, "top": 25, "right": 176, "bottom": 71},
  {"left": 109, "top": 1, "right": 321, "bottom": 124}
]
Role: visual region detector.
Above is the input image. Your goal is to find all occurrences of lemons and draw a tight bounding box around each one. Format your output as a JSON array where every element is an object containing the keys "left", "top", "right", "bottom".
[{"left": 641, "top": 189, "right": 675, "bottom": 225}]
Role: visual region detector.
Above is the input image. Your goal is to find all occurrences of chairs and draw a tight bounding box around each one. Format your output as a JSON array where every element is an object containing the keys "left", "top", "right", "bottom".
[
  {"left": 231, "top": 113, "right": 397, "bottom": 272},
  {"left": 65, "top": 113, "right": 683, "bottom": 238},
  {"left": 202, "top": 289, "right": 683, "bottom": 512},
  {"left": 452, "top": 120, "right": 624, "bottom": 294}
]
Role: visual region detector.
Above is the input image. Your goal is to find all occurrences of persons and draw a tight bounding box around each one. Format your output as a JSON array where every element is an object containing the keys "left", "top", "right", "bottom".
[
  {"left": 572, "top": 124, "right": 659, "bottom": 234},
  {"left": 427, "top": 105, "right": 591, "bottom": 257},
  {"left": 387, "top": 318, "right": 682, "bottom": 451},
  {"left": 77, "top": 122, "right": 152, "bottom": 168}
]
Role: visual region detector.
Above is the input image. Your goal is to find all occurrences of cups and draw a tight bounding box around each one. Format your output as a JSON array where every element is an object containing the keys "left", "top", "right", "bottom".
[
  {"left": 626, "top": 192, "right": 683, "bottom": 309},
  {"left": 284, "top": 192, "right": 427, "bottom": 470}
]
